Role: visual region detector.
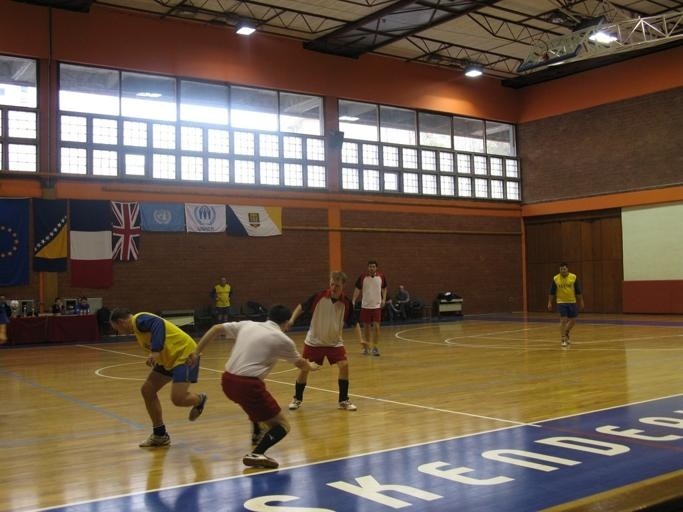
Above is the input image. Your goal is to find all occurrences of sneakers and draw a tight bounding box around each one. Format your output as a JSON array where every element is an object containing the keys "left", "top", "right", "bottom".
[
  {"left": 360, "top": 345, "right": 381, "bottom": 356},
  {"left": 242, "top": 452, "right": 279, "bottom": 469},
  {"left": 251, "top": 430, "right": 268, "bottom": 445},
  {"left": 337, "top": 399, "right": 358, "bottom": 411},
  {"left": 560, "top": 335, "right": 571, "bottom": 347},
  {"left": 138, "top": 431, "right": 172, "bottom": 448},
  {"left": 187, "top": 392, "right": 208, "bottom": 422},
  {"left": 288, "top": 396, "right": 304, "bottom": 410}
]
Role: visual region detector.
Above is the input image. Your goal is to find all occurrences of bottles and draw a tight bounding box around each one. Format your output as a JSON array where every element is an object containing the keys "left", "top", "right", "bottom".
[{"left": 23, "top": 304, "right": 35, "bottom": 317}]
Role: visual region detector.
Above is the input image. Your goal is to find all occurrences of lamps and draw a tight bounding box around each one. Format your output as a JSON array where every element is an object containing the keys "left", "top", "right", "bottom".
[
  {"left": 578, "top": 16, "right": 620, "bottom": 48},
  {"left": 232, "top": 19, "right": 256, "bottom": 38},
  {"left": 461, "top": 65, "right": 484, "bottom": 79}
]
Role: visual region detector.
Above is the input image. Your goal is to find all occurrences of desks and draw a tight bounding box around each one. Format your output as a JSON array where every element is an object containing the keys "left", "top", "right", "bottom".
[{"left": 2, "top": 314, "right": 103, "bottom": 347}]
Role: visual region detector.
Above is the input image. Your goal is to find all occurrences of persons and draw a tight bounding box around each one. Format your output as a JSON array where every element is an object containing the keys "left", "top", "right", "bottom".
[
  {"left": 352, "top": 259, "right": 387, "bottom": 356},
  {"left": 47, "top": 297, "right": 64, "bottom": 314},
  {"left": 284, "top": 270, "right": 372, "bottom": 411},
  {"left": 108, "top": 307, "right": 206, "bottom": 447},
  {"left": 395, "top": 284, "right": 412, "bottom": 321},
  {"left": 383, "top": 293, "right": 401, "bottom": 322},
  {"left": 73, "top": 294, "right": 89, "bottom": 315},
  {"left": 209, "top": 277, "right": 231, "bottom": 335},
  {"left": 546, "top": 262, "right": 585, "bottom": 346},
  {"left": 0, "top": 298, "right": 12, "bottom": 346},
  {"left": 184, "top": 304, "right": 320, "bottom": 468}
]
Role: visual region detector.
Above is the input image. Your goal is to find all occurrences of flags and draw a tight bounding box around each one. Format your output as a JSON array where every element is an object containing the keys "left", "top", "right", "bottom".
[
  {"left": 226, "top": 203, "right": 281, "bottom": 236},
  {"left": 184, "top": 202, "right": 227, "bottom": 234},
  {"left": 110, "top": 202, "right": 139, "bottom": 262},
  {"left": 138, "top": 200, "right": 185, "bottom": 233},
  {"left": 68, "top": 198, "right": 112, "bottom": 289},
  {"left": 31, "top": 195, "right": 69, "bottom": 273},
  {"left": 0, "top": 196, "right": 30, "bottom": 289}
]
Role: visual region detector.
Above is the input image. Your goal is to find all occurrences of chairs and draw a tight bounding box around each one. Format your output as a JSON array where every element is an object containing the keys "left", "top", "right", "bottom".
[{"left": 192, "top": 297, "right": 426, "bottom": 332}]
